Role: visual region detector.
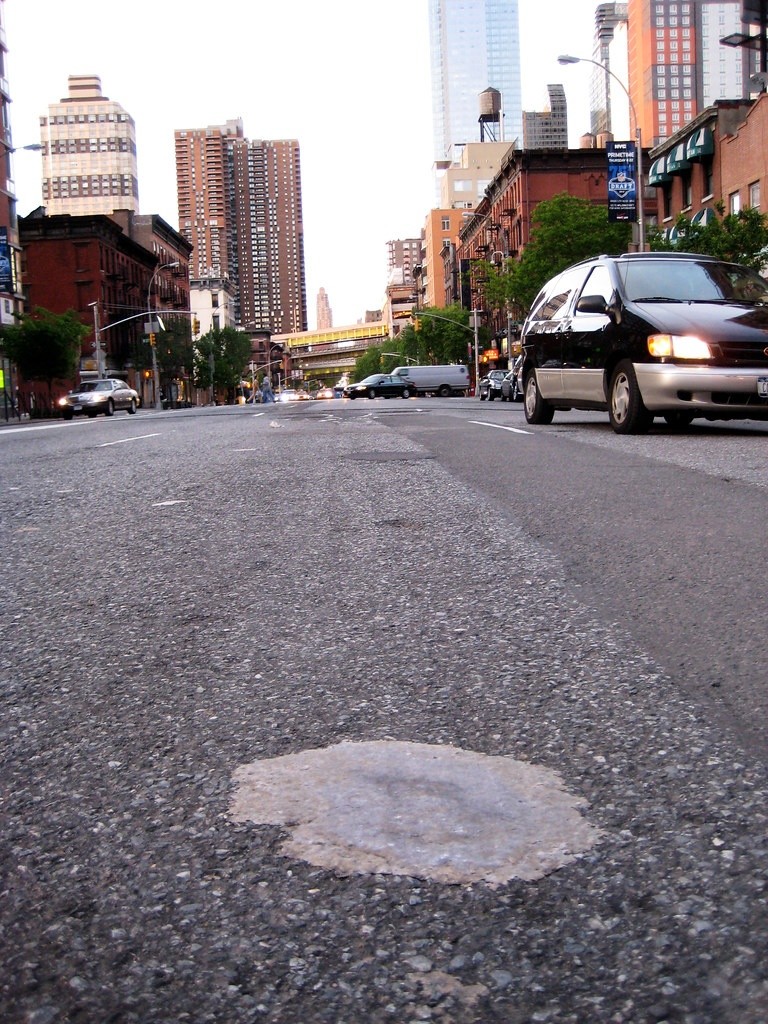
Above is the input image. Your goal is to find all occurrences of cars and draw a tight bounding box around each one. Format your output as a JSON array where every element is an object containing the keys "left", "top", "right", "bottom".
[
  {"left": 478, "top": 369, "right": 511, "bottom": 401},
  {"left": 57, "top": 378, "right": 141, "bottom": 420},
  {"left": 342, "top": 374, "right": 418, "bottom": 400},
  {"left": 274, "top": 386, "right": 345, "bottom": 403}
]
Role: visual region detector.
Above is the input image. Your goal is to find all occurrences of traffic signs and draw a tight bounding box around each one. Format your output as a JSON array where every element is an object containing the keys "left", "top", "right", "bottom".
[
  {"left": 470, "top": 345, "right": 485, "bottom": 350},
  {"left": 89, "top": 341, "right": 107, "bottom": 348},
  {"left": 502, "top": 328, "right": 517, "bottom": 335}
]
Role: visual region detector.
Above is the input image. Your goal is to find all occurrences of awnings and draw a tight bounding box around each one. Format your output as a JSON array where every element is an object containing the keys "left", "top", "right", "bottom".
[
  {"left": 649, "top": 156, "right": 673, "bottom": 187},
  {"left": 667, "top": 143, "right": 692, "bottom": 174},
  {"left": 662, "top": 208, "right": 715, "bottom": 244},
  {"left": 687, "top": 127, "right": 713, "bottom": 162}
]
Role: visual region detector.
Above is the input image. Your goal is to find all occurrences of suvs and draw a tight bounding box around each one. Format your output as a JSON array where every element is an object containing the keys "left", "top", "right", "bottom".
[{"left": 518, "top": 249, "right": 768, "bottom": 436}]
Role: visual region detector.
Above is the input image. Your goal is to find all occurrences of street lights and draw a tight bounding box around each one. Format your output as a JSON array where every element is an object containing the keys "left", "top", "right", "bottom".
[
  {"left": 147, "top": 260, "right": 180, "bottom": 410},
  {"left": 268, "top": 344, "right": 284, "bottom": 390},
  {"left": 460, "top": 211, "right": 512, "bottom": 371},
  {"left": 557, "top": 54, "right": 647, "bottom": 252},
  {"left": 208, "top": 301, "right": 236, "bottom": 406},
  {"left": 232, "top": 320, "right": 257, "bottom": 405}
]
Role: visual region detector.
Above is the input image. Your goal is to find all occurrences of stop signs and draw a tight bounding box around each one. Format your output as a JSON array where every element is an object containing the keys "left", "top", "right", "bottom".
[{"left": 183, "top": 374, "right": 190, "bottom": 381}]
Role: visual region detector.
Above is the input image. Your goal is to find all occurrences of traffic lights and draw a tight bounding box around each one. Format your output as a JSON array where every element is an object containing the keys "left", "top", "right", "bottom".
[
  {"left": 414, "top": 318, "right": 423, "bottom": 333},
  {"left": 144, "top": 370, "right": 152, "bottom": 378},
  {"left": 149, "top": 333, "right": 156, "bottom": 346},
  {"left": 246, "top": 383, "right": 252, "bottom": 389},
  {"left": 192, "top": 319, "right": 200, "bottom": 334},
  {"left": 481, "top": 355, "right": 489, "bottom": 365}
]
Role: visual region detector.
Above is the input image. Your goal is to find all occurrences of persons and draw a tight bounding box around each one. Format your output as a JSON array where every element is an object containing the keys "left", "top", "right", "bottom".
[
  {"left": 246, "top": 376, "right": 264, "bottom": 403},
  {"left": 261, "top": 374, "right": 276, "bottom": 403}
]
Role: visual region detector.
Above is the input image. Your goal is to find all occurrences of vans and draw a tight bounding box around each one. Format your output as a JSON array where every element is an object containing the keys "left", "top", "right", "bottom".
[
  {"left": 388, "top": 364, "right": 470, "bottom": 397},
  {"left": 500, "top": 354, "right": 524, "bottom": 403}
]
